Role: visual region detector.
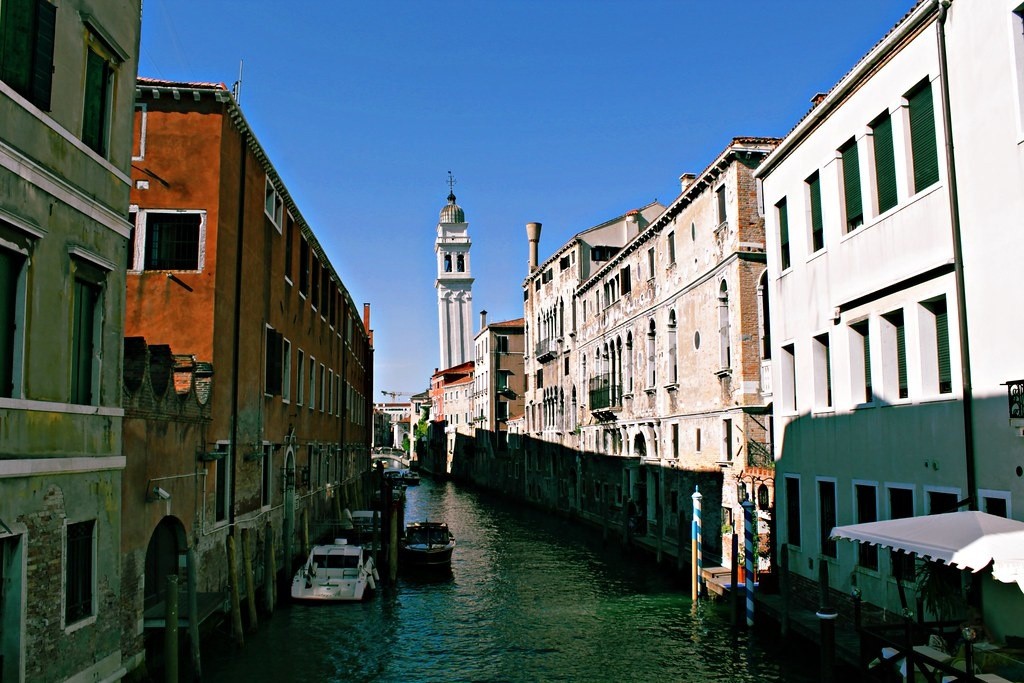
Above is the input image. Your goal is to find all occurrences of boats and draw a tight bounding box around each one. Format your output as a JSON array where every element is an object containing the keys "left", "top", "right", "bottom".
[
  {"left": 351, "top": 510, "right": 381, "bottom": 538},
  {"left": 385, "top": 469, "right": 420, "bottom": 504},
  {"left": 290, "top": 537, "right": 378, "bottom": 603},
  {"left": 400, "top": 516, "right": 456, "bottom": 566}
]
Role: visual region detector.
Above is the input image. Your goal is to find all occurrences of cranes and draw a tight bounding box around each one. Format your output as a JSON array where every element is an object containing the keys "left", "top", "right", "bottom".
[{"left": 381, "top": 389, "right": 419, "bottom": 401}]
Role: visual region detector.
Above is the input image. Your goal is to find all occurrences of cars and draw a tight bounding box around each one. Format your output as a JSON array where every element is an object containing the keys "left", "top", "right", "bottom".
[{"left": 372, "top": 446, "right": 410, "bottom": 468}]
